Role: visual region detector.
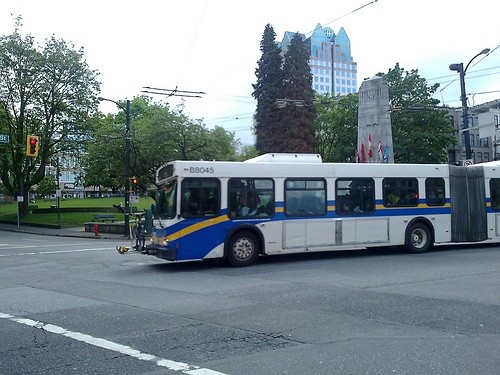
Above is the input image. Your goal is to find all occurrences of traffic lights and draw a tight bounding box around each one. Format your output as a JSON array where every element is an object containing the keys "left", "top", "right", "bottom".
[{"left": 30, "top": 137, "right": 38, "bottom": 154}]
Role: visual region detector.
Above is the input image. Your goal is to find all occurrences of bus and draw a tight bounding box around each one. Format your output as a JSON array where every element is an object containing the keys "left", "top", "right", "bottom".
[{"left": 142, "top": 159, "right": 500, "bottom": 266}]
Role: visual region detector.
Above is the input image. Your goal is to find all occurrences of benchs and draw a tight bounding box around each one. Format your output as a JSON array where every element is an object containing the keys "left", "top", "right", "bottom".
[{"left": 92, "top": 214, "right": 118, "bottom": 223}]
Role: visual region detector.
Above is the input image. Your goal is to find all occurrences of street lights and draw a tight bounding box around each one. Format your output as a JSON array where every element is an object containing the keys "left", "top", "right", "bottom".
[
  {"left": 449, "top": 46, "right": 492, "bottom": 164},
  {"left": 97, "top": 96, "right": 131, "bottom": 237}
]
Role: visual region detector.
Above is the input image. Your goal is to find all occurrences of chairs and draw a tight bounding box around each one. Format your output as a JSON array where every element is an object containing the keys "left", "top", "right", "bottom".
[{"left": 113, "top": 204, "right": 122, "bottom": 213}]
